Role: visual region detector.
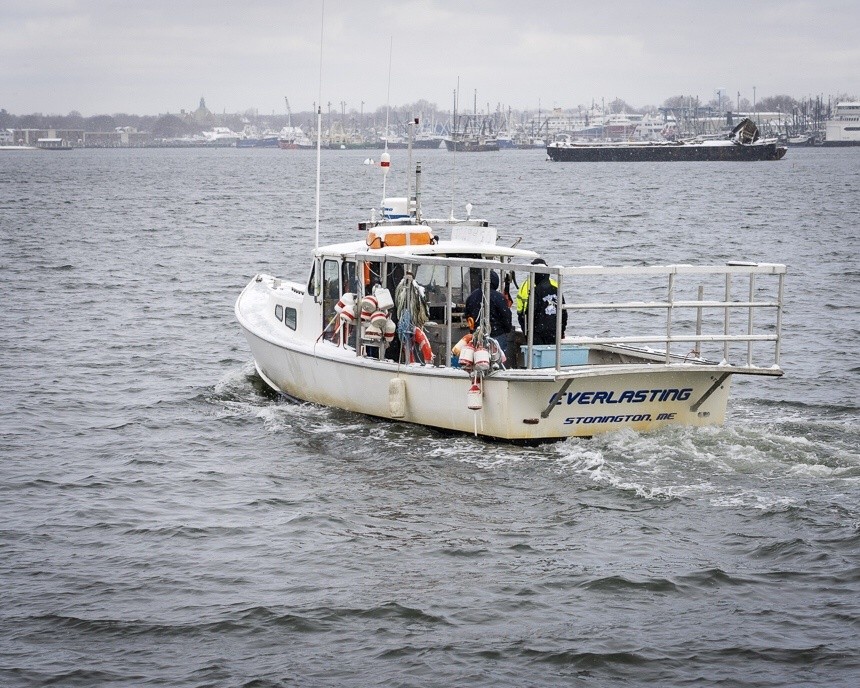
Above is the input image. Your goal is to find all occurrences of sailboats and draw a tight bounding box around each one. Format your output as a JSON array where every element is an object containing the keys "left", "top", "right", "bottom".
[{"left": 274, "top": 85, "right": 823, "bottom": 153}]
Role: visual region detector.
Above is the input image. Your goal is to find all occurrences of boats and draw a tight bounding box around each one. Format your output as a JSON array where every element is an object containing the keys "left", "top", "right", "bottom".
[
  {"left": 547, "top": 118, "right": 788, "bottom": 163},
  {"left": 233, "top": 35, "right": 791, "bottom": 443},
  {"left": 823, "top": 99, "right": 860, "bottom": 146},
  {"left": 38, "top": 138, "right": 73, "bottom": 150}
]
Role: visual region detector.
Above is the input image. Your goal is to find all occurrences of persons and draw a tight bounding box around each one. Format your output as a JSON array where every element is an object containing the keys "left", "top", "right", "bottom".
[
  {"left": 516, "top": 259, "right": 568, "bottom": 345},
  {"left": 465, "top": 270, "right": 512, "bottom": 351}
]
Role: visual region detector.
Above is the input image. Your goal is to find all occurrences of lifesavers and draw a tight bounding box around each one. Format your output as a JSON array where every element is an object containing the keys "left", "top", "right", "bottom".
[{"left": 404, "top": 326, "right": 435, "bottom": 367}]
